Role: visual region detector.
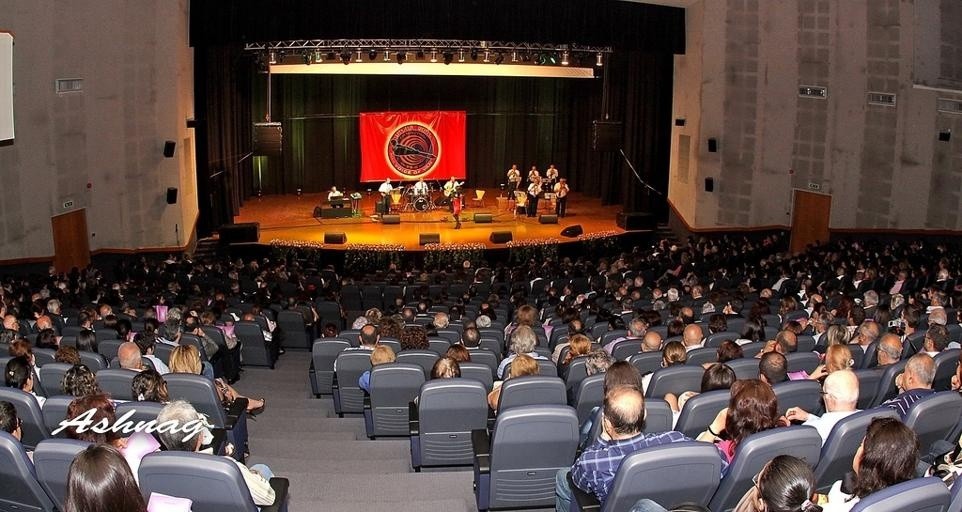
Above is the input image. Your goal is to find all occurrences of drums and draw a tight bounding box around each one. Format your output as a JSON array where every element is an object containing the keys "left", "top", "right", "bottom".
[{"left": 413, "top": 197, "right": 429, "bottom": 212}]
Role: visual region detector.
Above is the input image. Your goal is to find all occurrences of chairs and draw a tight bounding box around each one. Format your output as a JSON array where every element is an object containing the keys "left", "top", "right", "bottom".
[{"left": 1, "top": 227, "right": 962, "bottom": 510}]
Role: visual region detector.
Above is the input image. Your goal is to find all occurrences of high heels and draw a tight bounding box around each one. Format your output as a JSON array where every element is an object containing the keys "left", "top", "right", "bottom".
[{"left": 246, "top": 398, "right": 265, "bottom": 418}]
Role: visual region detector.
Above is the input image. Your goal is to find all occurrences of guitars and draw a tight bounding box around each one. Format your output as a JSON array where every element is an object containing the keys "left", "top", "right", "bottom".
[{"left": 444, "top": 181, "right": 464, "bottom": 197}]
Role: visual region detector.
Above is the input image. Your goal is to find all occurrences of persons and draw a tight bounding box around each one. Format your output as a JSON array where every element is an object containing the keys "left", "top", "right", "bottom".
[
  {"left": 527, "top": 164, "right": 540, "bottom": 183},
  {"left": 444, "top": 176, "right": 464, "bottom": 199},
  {"left": 524, "top": 176, "right": 542, "bottom": 218},
  {"left": 329, "top": 186, "right": 343, "bottom": 208},
  {"left": 379, "top": 179, "right": 395, "bottom": 217},
  {"left": 410, "top": 177, "right": 430, "bottom": 198},
  {"left": 507, "top": 164, "right": 520, "bottom": 198},
  {"left": 447, "top": 187, "right": 466, "bottom": 228},
  {"left": 554, "top": 176, "right": 569, "bottom": 219},
  {"left": 546, "top": 164, "right": 560, "bottom": 189}
]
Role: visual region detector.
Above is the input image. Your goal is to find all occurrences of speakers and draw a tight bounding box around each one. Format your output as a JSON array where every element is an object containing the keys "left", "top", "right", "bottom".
[
  {"left": 163, "top": 140, "right": 176, "bottom": 157},
  {"left": 616, "top": 211, "right": 657, "bottom": 231},
  {"left": 675, "top": 119, "right": 684, "bottom": 126},
  {"left": 188, "top": 120, "right": 199, "bottom": 128},
  {"left": 220, "top": 223, "right": 260, "bottom": 242},
  {"left": 255, "top": 123, "right": 281, "bottom": 156},
  {"left": 709, "top": 137, "right": 716, "bottom": 152},
  {"left": 324, "top": 213, "right": 583, "bottom": 246},
  {"left": 705, "top": 177, "right": 713, "bottom": 191},
  {"left": 167, "top": 187, "right": 177, "bottom": 204},
  {"left": 375, "top": 201, "right": 383, "bottom": 212},
  {"left": 592, "top": 121, "right": 622, "bottom": 152}
]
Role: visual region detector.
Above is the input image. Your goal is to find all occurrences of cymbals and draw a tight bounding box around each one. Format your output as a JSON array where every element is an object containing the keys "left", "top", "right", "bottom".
[
  {"left": 426, "top": 182, "right": 434, "bottom": 185},
  {"left": 426, "top": 189, "right": 437, "bottom": 192}
]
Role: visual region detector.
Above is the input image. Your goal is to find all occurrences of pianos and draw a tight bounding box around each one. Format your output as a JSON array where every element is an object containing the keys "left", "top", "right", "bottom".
[{"left": 328, "top": 196, "right": 351, "bottom": 205}]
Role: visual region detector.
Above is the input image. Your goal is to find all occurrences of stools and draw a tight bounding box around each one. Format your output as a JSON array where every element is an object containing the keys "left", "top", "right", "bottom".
[{"left": 471, "top": 198, "right": 485, "bottom": 207}]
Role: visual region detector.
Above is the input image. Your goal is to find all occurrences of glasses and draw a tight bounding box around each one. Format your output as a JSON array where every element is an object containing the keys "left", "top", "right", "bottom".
[{"left": 751, "top": 472, "right": 764, "bottom": 499}]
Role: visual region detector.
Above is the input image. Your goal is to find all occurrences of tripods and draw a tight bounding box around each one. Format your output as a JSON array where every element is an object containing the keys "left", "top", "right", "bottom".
[{"left": 403, "top": 181, "right": 437, "bottom": 211}]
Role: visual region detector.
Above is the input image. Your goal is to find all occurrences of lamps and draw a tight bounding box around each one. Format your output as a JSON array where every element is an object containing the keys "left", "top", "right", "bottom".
[{"left": 245, "top": 38, "right": 615, "bottom": 67}]
